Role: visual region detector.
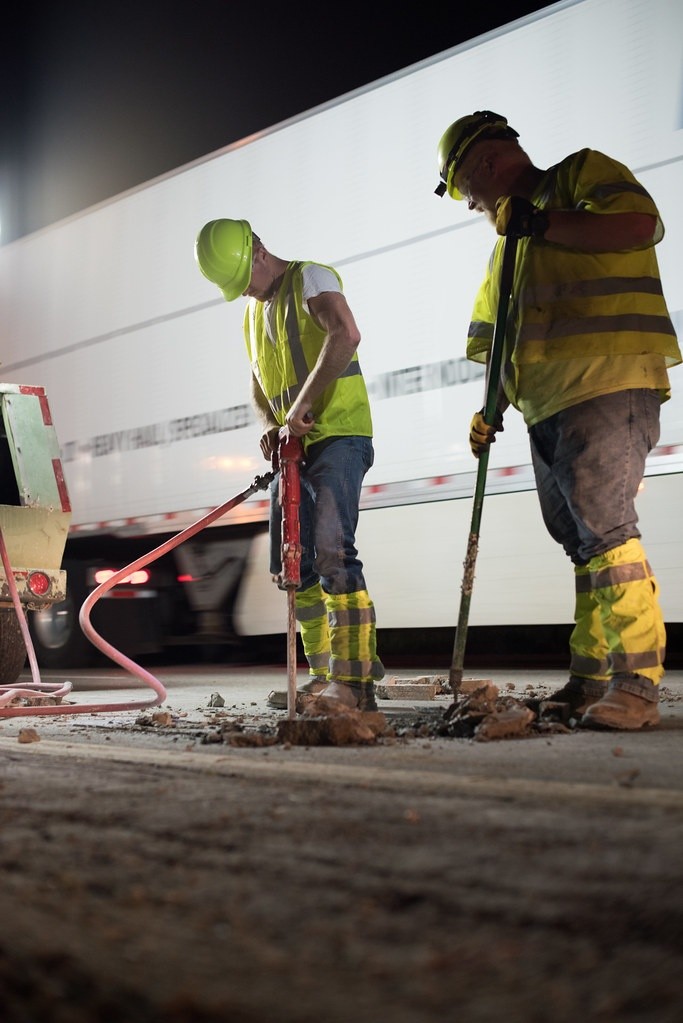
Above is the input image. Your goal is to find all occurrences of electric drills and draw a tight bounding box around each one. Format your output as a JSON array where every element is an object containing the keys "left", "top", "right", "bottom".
[{"left": 271, "top": 410, "right": 313, "bottom": 719}]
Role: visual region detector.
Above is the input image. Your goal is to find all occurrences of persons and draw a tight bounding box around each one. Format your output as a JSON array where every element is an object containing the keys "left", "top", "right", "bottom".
[
  {"left": 438, "top": 112, "right": 683, "bottom": 730},
  {"left": 194, "top": 218, "right": 386, "bottom": 714}
]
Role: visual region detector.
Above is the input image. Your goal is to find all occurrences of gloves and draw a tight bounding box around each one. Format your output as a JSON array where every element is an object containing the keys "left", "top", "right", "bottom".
[
  {"left": 495, "top": 195, "right": 550, "bottom": 244},
  {"left": 469, "top": 405, "right": 504, "bottom": 458}
]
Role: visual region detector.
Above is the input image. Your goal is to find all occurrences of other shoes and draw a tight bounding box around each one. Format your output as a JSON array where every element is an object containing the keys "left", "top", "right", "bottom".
[
  {"left": 543, "top": 686, "right": 605, "bottom": 716},
  {"left": 265, "top": 679, "right": 328, "bottom": 709},
  {"left": 294, "top": 682, "right": 378, "bottom": 716},
  {"left": 582, "top": 688, "right": 661, "bottom": 729}
]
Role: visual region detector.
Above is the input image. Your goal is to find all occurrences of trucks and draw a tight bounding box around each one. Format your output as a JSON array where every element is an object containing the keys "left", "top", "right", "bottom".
[{"left": 0, "top": 382, "right": 72, "bottom": 682}]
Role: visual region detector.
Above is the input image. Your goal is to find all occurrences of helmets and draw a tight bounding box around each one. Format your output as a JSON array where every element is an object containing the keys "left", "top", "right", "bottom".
[
  {"left": 193, "top": 218, "right": 252, "bottom": 302},
  {"left": 437, "top": 110, "right": 520, "bottom": 201}
]
